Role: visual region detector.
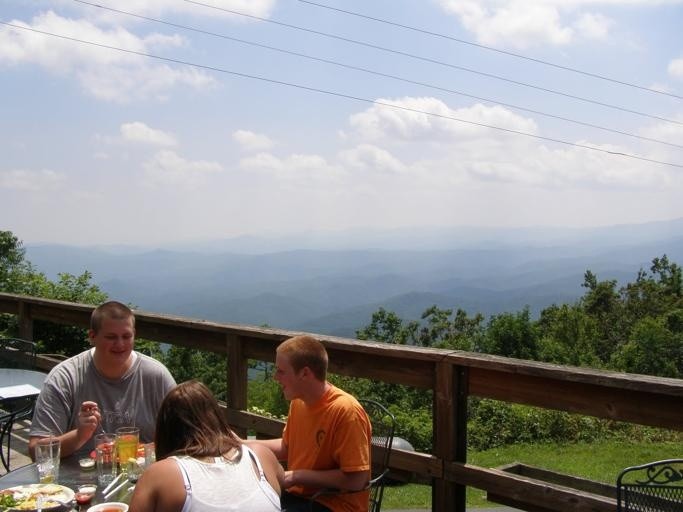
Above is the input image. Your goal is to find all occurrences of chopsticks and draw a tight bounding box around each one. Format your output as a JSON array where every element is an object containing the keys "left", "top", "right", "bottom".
[{"left": 102, "top": 472, "right": 129, "bottom": 499}]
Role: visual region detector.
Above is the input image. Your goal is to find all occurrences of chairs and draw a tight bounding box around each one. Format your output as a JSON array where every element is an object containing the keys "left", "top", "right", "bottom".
[
  {"left": 0, "top": 338, "right": 37, "bottom": 472},
  {"left": 280, "top": 399, "right": 397, "bottom": 512},
  {"left": 616, "top": 458, "right": 682, "bottom": 512}
]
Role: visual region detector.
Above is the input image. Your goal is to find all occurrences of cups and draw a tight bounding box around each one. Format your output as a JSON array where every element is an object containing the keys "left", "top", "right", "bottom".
[{"left": 33, "top": 426, "right": 157, "bottom": 483}]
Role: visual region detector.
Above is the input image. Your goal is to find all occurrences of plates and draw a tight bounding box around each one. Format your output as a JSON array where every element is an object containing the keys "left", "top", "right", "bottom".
[{"left": 0, "top": 483, "right": 75, "bottom": 512}]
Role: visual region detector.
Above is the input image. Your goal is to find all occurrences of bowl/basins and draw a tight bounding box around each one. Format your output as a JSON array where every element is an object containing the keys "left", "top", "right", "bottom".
[
  {"left": 85, "top": 502, "right": 128, "bottom": 512},
  {"left": 79, "top": 484, "right": 98, "bottom": 493},
  {"left": 76, "top": 493, "right": 96, "bottom": 503}
]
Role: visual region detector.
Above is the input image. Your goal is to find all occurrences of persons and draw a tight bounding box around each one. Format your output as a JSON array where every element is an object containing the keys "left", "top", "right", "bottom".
[
  {"left": 29, "top": 301, "right": 178, "bottom": 463},
  {"left": 128, "top": 380, "right": 285, "bottom": 512},
  {"left": 228, "top": 335, "right": 372, "bottom": 512}
]
